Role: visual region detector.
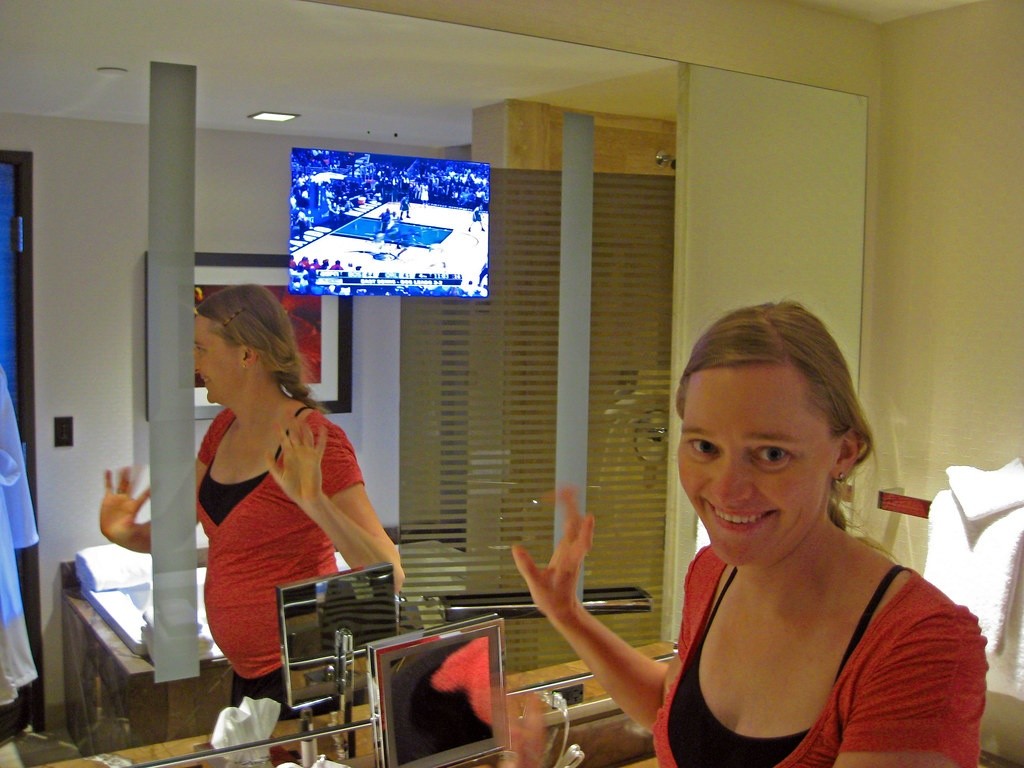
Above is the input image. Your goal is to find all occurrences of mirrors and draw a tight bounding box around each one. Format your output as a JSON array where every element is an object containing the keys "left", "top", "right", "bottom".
[{"left": 0, "top": 1, "right": 679, "bottom": 766}]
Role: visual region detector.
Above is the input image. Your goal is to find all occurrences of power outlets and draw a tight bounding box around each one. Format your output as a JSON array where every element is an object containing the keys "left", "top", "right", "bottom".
[{"left": 55, "top": 418, "right": 73, "bottom": 446}]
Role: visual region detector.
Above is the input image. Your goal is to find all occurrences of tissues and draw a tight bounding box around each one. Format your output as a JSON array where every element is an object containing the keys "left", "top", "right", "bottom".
[{"left": 192, "top": 695, "right": 296, "bottom": 768}]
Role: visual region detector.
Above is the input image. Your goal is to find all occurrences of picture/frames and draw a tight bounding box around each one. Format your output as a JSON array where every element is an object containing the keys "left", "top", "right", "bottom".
[{"left": 142, "top": 248, "right": 354, "bottom": 420}]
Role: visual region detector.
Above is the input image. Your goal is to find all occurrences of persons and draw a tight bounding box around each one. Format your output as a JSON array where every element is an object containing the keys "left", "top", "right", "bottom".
[
  {"left": 507, "top": 299, "right": 988, "bottom": 767},
  {"left": 288, "top": 148, "right": 488, "bottom": 296},
  {"left": 102, "top": 285, "right": 406, "bottom": 720}
]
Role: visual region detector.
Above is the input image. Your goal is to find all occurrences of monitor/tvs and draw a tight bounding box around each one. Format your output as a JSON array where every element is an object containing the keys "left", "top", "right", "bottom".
[{"left": 289, "top": 148, "right": 491, "bottom": 298}]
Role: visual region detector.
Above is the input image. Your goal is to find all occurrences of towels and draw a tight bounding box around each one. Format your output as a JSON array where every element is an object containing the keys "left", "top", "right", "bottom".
[{"left": 922, "top": 458, "right": 1024, "bottom": 700}]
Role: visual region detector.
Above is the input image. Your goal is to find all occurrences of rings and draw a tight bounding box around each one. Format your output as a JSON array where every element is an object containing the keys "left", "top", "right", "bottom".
[{"left": 119, "top": 490, "right": 127, "bottom": 493}]
